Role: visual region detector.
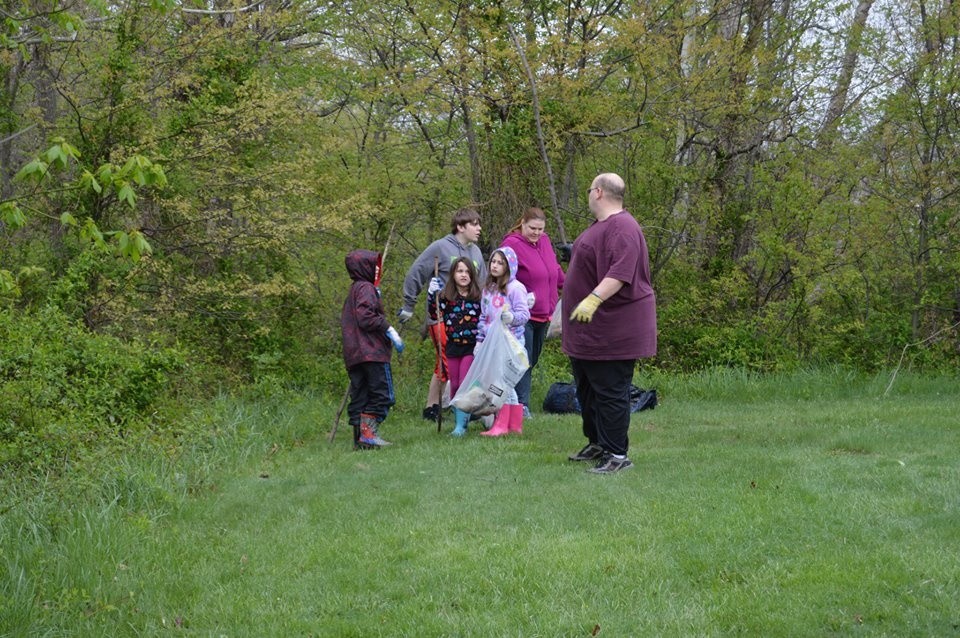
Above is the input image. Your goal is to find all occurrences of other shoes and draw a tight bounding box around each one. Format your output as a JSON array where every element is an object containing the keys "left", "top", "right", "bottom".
[
  {"left": 423, "top": 404, "right": 447, "bottom": 422},
  {"left": 523, "top": 407, "right": 533, "bottom": 417}
]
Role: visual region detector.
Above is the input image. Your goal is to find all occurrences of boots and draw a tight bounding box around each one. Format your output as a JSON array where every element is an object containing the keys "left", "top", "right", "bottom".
[
  {"left": 481, "top": 405, "right": 509, "bottom": 435},
  {"left": 353, "top": 424, "right": 365, "bottom": 449},
  {"left": 510, "top": 405, "right": 523, "bottom": 432},
  {"left": 359, "top": 414, "right": 393, "bottom": 446},
  {"left": 450, "top": 409, "right": 471, "bottom": 437}
]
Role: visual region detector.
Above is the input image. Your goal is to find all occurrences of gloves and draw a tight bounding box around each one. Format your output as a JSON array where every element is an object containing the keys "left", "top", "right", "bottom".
[
  {"left": 556, "top": 243, "right": 572, "bottom": 262},
  {"left": 569, "top": 293, "right": 604, "bottom": 323},
  {"left": 527, "top": 293, "right": 535, "bottom": 308},
  {"left": 396, "top": 310, "right": 413, "bottom": 323},
  {"left": 502, "top": 311, "right": 514, "bottom": 324},
  {"left": 386, "top": 326, "right": 404, "bottom": 352},
  {"left": 428, "top": 278, "right": 444, "bottom": 294},
  {"left": 473, "top": 342, "right": 482, "bottom": 356}
]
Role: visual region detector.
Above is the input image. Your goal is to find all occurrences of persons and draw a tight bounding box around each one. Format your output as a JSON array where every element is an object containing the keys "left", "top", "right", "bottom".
[
  {"left": 557, "top": 172, "right": 657, "bottom": 473},
  {"left": 398, "top": 207, "right": 487, "bottom": 421},
  {"left": 473, "top": 245, "right": 530, "bottom": 435},
  {"left": 427, "top": 256, "right": 481, "bottom": 434},
  {"left": 341, "top": 249, "right": 405, "bottom": 451},
  {"left": 499, "top": 206, "right": 564, "bottom": 417}
]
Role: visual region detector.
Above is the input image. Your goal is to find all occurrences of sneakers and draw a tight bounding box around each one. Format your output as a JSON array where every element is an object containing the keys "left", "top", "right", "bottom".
[
  {"left": 569, "top": 446, "right": 607, "bottom": 460},
  {"left": 586, "top": 455, "right": 634, "bottom": 473}
]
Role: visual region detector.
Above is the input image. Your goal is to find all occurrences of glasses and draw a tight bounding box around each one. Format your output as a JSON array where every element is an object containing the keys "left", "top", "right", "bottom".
[{"left": 588, "top": 188, "right": 598, "bottom": 193}]
